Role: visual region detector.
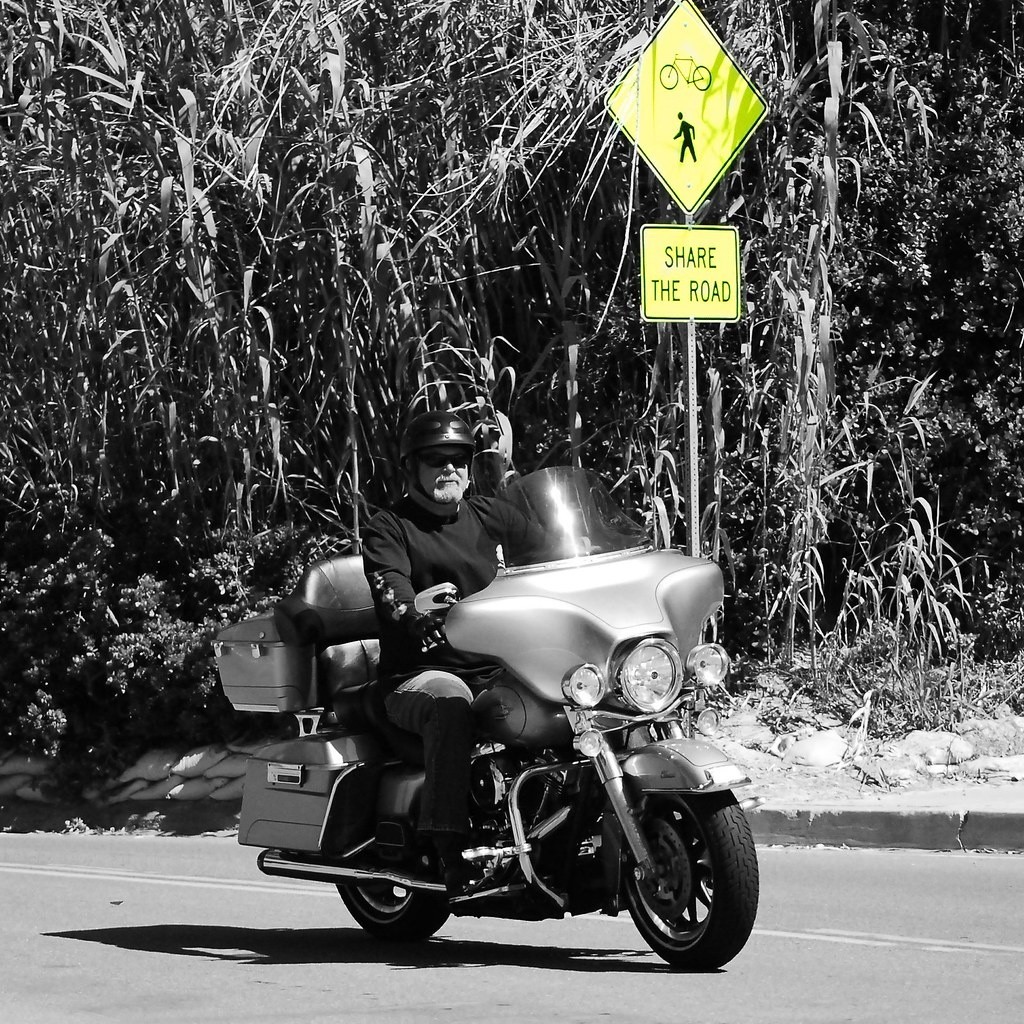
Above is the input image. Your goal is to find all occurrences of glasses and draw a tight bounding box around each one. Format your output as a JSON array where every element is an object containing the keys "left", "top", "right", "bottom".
[{"left": 416, "top": 447, "right": 472, "bottom": 469}]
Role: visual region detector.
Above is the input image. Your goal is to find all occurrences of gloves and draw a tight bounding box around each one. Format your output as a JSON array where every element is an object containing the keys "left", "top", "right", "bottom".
[{"left": 395, "top": 606, "right": 444, "bottom": 654}]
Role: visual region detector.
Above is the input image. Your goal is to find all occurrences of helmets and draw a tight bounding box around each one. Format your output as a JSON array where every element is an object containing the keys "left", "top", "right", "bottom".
[{"left": 401, "top": 412, "right": 481, "bottom": 466}]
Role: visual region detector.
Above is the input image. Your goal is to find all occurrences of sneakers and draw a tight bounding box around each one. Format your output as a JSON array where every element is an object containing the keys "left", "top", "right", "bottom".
[{"left": 415, "top": 839, "right": 484, "bottom": 883}]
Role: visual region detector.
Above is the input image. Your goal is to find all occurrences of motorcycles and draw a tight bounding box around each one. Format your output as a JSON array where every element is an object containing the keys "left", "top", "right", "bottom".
[{"left": 213, "top": 462, "right": 764, "bottom": 973}]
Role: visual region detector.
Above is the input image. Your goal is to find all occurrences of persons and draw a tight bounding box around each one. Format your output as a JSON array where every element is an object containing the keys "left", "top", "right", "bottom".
[{"left": 362, "top": 411, "right": 561, "bottom": 904}]
